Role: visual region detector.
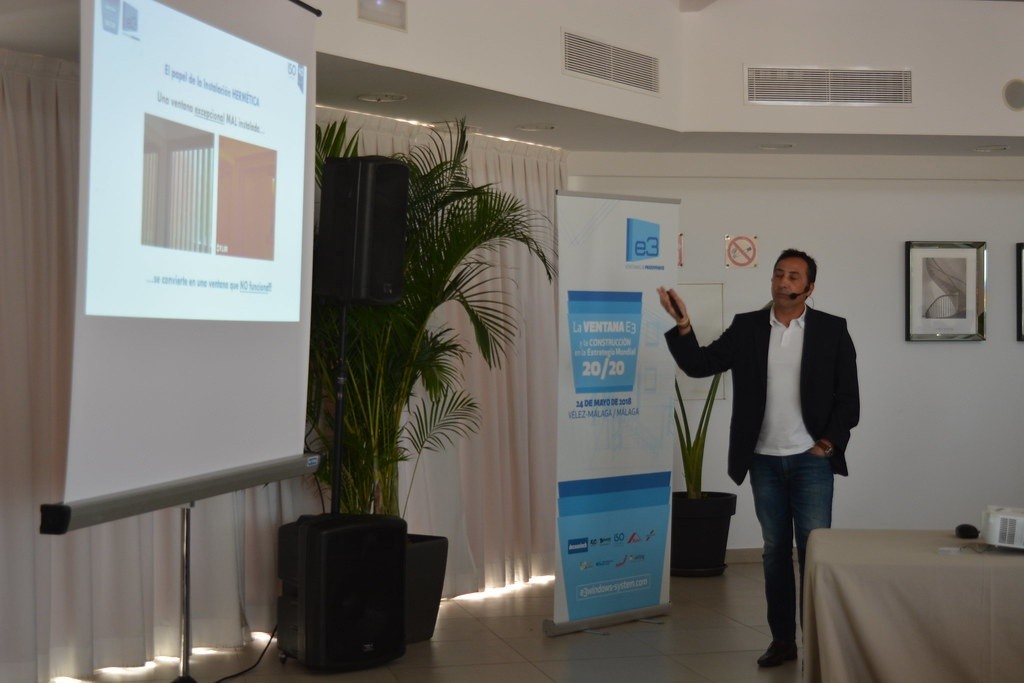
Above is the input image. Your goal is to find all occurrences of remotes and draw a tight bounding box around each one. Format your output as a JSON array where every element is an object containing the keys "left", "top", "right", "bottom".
[{"left": 666, "top": 291, "right": 683, "bottom": 318}]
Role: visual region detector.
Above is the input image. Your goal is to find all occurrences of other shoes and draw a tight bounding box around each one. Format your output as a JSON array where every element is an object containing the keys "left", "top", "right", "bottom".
[{"left": 757, "top": 640, "right": 798, "bottom": 666}]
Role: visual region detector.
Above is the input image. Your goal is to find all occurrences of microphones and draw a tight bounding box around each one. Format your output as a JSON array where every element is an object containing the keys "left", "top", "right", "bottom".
[{"left": 790, "top": 286, "right": 809, "bottom": 300}]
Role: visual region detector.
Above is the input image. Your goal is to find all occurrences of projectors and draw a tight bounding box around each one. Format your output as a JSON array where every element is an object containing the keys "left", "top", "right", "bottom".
[{"left": 980, "top": 506, "right": 1024, "bottom": 549}]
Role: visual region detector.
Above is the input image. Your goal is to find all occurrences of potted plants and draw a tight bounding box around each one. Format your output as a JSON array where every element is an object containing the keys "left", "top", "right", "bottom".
[
  {"left": 278, "top": 114, "right": 560, "bottom": 645},
  {"left": 670, "top": 372, "right": 737, "bottom": 578}
]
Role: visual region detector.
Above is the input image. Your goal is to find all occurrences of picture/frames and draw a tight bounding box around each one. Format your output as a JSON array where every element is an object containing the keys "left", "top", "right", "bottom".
[
  {"left": 904, "top": 241, "right": 987, "bottom": 340},
  {"left": 1016, "top": 243, "right": 1024, "bottom": 342}
]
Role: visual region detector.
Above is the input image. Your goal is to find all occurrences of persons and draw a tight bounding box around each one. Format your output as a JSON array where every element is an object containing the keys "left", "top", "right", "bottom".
[{"left": 657, "top": 248, "right": 860, "bottom": 667}]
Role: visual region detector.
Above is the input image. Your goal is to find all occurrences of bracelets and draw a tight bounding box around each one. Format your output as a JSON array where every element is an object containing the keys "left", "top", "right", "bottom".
[{"left": 816, "top": 441, "right": 833, "bottom": 457}]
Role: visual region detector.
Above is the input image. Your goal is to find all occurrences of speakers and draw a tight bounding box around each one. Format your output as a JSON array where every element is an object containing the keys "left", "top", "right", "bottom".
[{"left": 277, "top": 512, "right": 407, "bottom": 672}]
